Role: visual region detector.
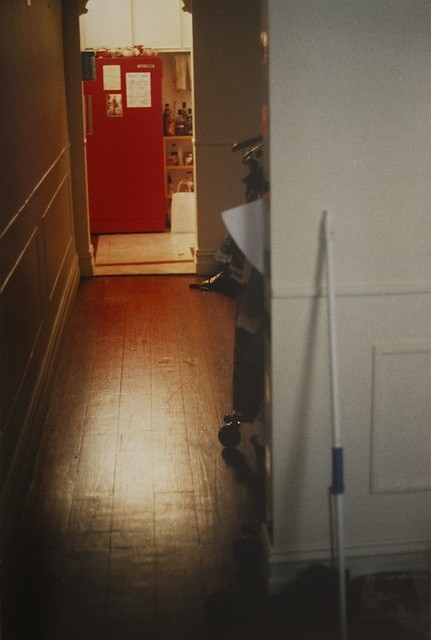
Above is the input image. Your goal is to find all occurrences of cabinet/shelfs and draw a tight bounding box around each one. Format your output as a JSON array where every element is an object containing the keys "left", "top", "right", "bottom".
[{"left": 163, "top": 99, "right": 196, "bottom": 231}]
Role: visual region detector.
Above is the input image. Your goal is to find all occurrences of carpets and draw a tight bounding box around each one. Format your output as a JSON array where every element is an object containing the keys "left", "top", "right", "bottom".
[{"left": 93, "top": 233, "right": 197, "bottom": 267}]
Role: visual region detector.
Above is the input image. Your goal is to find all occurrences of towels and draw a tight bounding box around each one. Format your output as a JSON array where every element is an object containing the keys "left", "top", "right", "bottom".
[{"left": 171, "top": 52, "right": 189, "bottom": 92}]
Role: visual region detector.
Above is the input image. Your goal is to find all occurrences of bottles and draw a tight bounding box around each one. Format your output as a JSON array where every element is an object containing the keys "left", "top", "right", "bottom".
[
  {"left": 171, "top": 143, "right": 178, "bottom": 165},
  {"left": 177, "top": 144, "right": 184, "bottom": 166},
  {"left": 164, "top": 101, "right": 193, "bottom": 136}
]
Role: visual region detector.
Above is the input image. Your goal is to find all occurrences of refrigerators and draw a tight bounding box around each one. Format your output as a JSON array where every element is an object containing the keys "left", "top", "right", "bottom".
[{"left": 83, "top": 56, "right": 167, "bottom": 234}]
